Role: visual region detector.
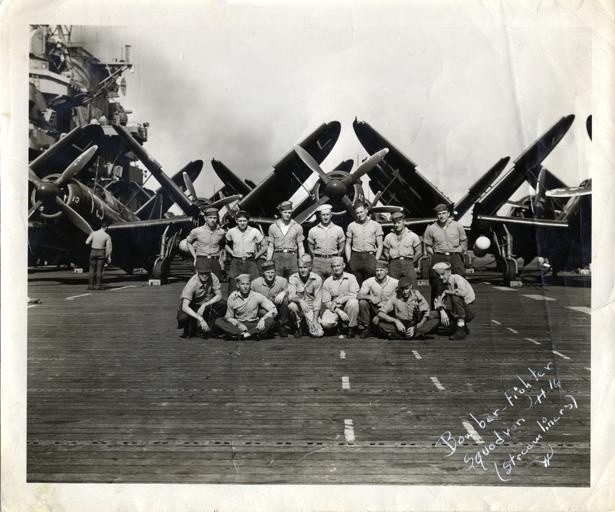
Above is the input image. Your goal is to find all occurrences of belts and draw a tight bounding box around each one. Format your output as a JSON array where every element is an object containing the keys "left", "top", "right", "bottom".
[
  {"left": 274, "top": 248, "right": 294, "bottom": 254},
  {"left": 314, "top": 252, "right": 338, "bottom": 258},
  {"left": 436, "top": 251, "right": 457, "bottom": 256},
  {"left": 197, "top": 255, "right": 220, "bottom": 259},
  {"left": 234, "top": 256, "right": 253, "bottom": 262},
  {"left": 394, "top": 256, "right": 412, "bottom": 261}
]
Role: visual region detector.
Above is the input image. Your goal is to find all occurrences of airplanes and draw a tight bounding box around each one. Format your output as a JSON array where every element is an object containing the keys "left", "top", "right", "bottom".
[{"left": 29, "top": 115, "right": 591, "bottom": 290}]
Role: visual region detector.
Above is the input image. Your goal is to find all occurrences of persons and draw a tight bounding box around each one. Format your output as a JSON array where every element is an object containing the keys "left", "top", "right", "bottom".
[
  {"left": 176, "top": 201, "right": 476, "bottom": 340},
  {"left": 85, "top": 223, "right": 113, "bottom": 289}
]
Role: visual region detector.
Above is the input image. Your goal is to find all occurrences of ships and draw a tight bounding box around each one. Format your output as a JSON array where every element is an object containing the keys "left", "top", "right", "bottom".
[{"left": 29, "top": 25, "right": 149, "bottom": 202}]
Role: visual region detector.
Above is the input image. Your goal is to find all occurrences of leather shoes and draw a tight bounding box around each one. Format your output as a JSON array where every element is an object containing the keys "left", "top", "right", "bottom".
[
  {"left": 294, "top": 326, "right": 303, "bottom": 337},
  {"left": 360, "top": 326, "right": 369, "bottom": 339},
  {"left": 345, "top": 327, "right": 354, "bottom": 337},
  {"left": 279, "top": 324, "right": 289, "bottom": 337},
  {"left": 227, "top": 332, "right": 245, "bottom": 341},
  {"left": 387, "top": 331, "right": 401, "bottom": 340},
  {"left": 250, "top": 332, "right": 261, "bottom": 341},
  {"left": 450, "top": 331, "right": 466, "bottom": 340}
]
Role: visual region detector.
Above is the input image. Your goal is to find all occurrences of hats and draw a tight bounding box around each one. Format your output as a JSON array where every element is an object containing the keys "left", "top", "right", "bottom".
[
  {"left": 435, "top": 204, "right": 447, "bottom": 212},
  {"left": 316, "top": 204, "right": 332, "bottom": 213},
  {"left": 276, "top": 201, "right": 293, "bottom": 211},
  {"left": 235, "top": 253, "right": 344, "bottom": 282},
  {"left": 376, "top": 260, "right": 388, "bottom": 268},
  {"left": 195, "top": 262, "right": 212, "bottom": 274},
  {"left": 235, "top": 210, "right": 249, "bottom": 219},
  {"left": 433, "top": 261, "right": 452, "bottom": 274},
  {"left": 389, "top": 212, "right": 403, "bottom": 222},
  {"left": 396, "top": 277, "right": 412, "bottom": 288},
  {"left": 203, "top": 208, "right": 220, "bottom": 217}
]
